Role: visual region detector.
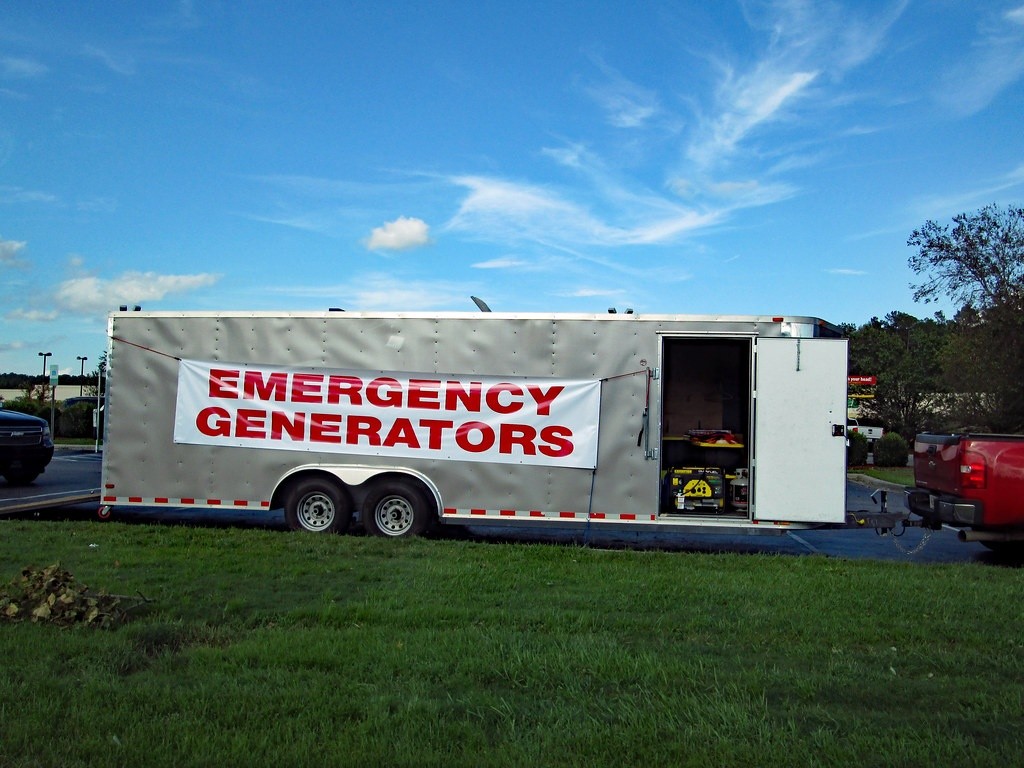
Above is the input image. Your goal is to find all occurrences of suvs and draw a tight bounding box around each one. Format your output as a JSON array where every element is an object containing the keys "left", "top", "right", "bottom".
[{"left": 0, "top": 408, "right": 54, "bottom": 484}]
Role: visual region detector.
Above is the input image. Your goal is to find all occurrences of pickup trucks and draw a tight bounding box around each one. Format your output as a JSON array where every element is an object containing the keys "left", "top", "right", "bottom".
[
  {"left": 903, "top": 431, "right": 1024, "bottom": 553},
  {"left": 846, "top": 417, "right": 883, "bottom": 447}
]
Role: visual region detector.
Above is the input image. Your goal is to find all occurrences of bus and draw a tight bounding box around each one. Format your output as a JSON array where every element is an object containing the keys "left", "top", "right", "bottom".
[{"left": 848, "top": 394, "right": 874, "bottom": 418}]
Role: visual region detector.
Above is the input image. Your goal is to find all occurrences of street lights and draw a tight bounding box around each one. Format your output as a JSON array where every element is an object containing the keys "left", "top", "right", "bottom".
[
  {"left": 77, "top": 356, "right": 87, "bottom": 395},
  {"left": 38, "top": 352, "right": 52, "bottom": 400}
]
